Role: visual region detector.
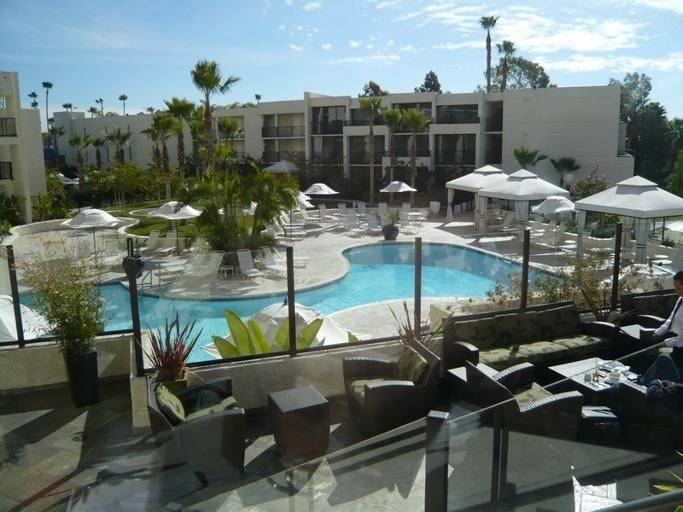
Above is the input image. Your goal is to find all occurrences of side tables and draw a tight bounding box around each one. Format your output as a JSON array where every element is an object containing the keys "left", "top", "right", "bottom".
[
  {"left": 618, "top": 327, "right": 656, "bottom": 351},
  {"left": 446, "top": 363, "right": 499, "bottom": 401}
]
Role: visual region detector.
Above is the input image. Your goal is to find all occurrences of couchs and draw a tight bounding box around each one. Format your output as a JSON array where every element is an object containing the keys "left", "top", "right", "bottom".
[
  {"left": 620, "top": 288, "right": 679, "bottom": 328},
  {"left": 442, "top": 300, "right": 618, "bottom": 376}
]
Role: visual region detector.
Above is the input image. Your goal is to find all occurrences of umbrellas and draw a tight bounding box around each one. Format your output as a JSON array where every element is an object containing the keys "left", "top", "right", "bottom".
[
  {"left": 218, "top": 199, "right": 258, "bottom": 224},
  {"left": 148, "top": 200, "right": 203, "bottom": 255},
  {"left": 294, "top": 182, "right": 340, "bottom": 220},
  {"left": 531, "top": 193, "right": 579, "bottom": 251},
  {"left": 0, "top": 295, "right": 59, "bottom": 345},
  {"left": 62, "top": 209, "right": 125, "bottom": 267},
  {"left": 380, "top": 180, "right": 418, "bottom": 218}
]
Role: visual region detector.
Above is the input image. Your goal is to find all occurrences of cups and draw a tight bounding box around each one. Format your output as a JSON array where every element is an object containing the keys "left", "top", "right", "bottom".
[{"left": 584, "top": 360, "right": 632, "bottom": 385}]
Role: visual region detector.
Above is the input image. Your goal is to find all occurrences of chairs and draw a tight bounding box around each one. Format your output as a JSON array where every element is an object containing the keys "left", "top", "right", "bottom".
[
  {"left": 144, "top": 374, "right": 247, "bottom": 490},
  {"left": 342, "top": 338, "right": 442, "bottom": 436},
  {"left": 474, "top": 208, "right": 634, "bottom": 267},
  {"left": 640, "top": 328, "right": 659, "bottom": 347},
  {"left": 465, "top": 360, "right": 584, "bottom": 443},
  {"left": 619, "top": 380, "right": 647, "bottom": 411}
]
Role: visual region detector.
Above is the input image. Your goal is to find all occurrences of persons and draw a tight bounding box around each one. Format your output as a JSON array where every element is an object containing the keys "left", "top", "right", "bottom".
[
  {"left": 634, "top": 354, "right": 683, "bottom": 411},
  {"left": 591, "top": 217, "right": 599, "bottom": 237},
  {"left": 652, "top": 271, "right": 683, "bottom": 364}
]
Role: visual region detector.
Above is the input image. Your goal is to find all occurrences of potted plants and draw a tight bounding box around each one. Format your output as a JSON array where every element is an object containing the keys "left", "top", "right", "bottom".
[
  {"left": 5, "top": 217, "right": 115, "bottom": 408},
  {"left": 134, "top": 309, "right": 206, "bottom": 394}
]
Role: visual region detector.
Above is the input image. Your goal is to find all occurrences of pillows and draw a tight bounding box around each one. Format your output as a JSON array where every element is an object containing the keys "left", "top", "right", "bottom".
[
  {"left": 155, "top": 382, "right": 185, "bottom": 426},
  {"left": 454, "top": 303, "right": 582, "bottom": 348},
  {"left": 393, "top": 345, "right": 428, "bottom": 384},
  {"left": 631, "top": 293, "right": 679, "bottom": 315}
]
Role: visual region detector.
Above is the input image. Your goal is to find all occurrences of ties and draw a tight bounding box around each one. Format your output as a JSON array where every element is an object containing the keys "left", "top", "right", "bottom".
[{"left": 666, "top": 298, "right": 682, "bottom": 330}]
